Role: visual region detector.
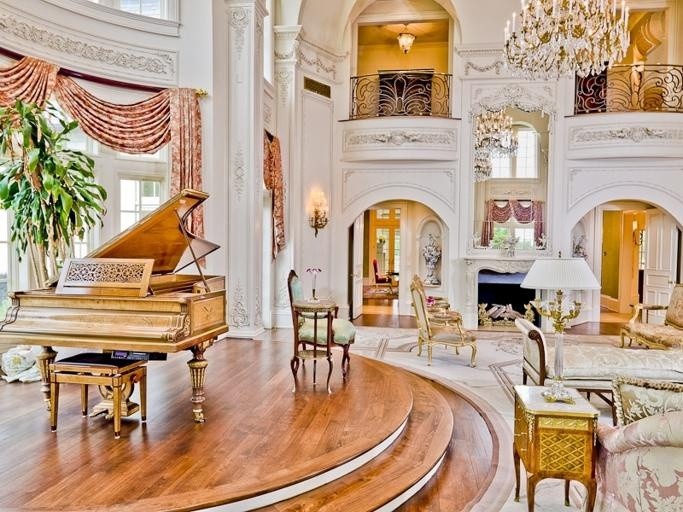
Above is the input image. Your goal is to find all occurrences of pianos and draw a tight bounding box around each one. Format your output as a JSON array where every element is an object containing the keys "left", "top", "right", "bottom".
[{"left": 0, "top": 188, "right": 229, "bottom": 424}]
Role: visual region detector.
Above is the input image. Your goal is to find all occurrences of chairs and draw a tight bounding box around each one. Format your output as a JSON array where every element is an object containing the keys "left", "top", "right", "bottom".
[
  {"left": 372, "top": 258, "right": 394, "bottom": 296},
  {"left": 514, "top": 316, "right": 618, "bottom": 428},
  {"left": 596, "top": 411, "right": 683, "bottom": 512},
  {"left": 287, "top": 269, "right": 356, "bottom": 384},
  {"left": 409, "top": 275, "right": 478, "bottom": 369},
  {"left": 618, "top": 283, "right": 683, "bottom": 350}
]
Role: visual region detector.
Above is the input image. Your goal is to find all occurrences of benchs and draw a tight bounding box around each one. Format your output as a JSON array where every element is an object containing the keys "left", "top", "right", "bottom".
[{"left": 514, "top": 317, "right": 683, "bottom": 407}]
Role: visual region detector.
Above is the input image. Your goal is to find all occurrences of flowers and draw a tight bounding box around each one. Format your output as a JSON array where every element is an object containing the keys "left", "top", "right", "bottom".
[{"left": 304, "top": 264, "right": 322, "bottom": 273}]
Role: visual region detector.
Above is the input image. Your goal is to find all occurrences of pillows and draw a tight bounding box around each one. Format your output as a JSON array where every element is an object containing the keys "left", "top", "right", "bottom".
[{"left": 613, "top": 374, "right": 683, "bottom": 425}]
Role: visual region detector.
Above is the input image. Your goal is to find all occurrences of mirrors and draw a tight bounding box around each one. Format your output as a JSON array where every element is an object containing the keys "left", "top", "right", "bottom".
[{"left": 467, "top": 84, "right": 555, "bottom": 261}]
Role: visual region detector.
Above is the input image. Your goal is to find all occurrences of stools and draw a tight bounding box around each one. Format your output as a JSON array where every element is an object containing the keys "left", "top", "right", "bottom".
[{"left": 48, "top": 353, "right": 147, "bottom": 440}]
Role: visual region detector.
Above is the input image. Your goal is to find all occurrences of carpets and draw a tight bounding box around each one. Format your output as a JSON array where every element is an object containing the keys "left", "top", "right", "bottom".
[{"left": 363, "top": 286, "right": 399, "bottom": 300}]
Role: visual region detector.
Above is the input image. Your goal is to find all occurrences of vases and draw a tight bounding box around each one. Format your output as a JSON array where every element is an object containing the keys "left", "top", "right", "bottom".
[{"left": 308, "top": 273, "right": 320, "bottom": 303}]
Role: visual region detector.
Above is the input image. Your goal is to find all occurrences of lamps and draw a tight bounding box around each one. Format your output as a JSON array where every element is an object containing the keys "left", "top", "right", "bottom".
[
  {"left": 520, "top": 254, "right": 602, "bottom": 405},
  {"left": 633, "top": 223, "right": 647, "bottom": 246},
  {"left": 472, "top": 108, "right": 519, "bottom": 181},
  {"left": 396, "top": 22, "right": 417, "bottom": 54},
  {"left": 307, "top": 189, "right": 328, "bottom": 238},
  {"left": 503, "top": 0, "right": 631, "bottom": 81}
]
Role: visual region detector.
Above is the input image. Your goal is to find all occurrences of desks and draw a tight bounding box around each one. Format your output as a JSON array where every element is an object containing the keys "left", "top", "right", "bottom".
[
  {"left": 386, "top": 271, "right": 399, "bottom": 277},
  {"left": 290, "top": 299, "right": 336, "bottom": 394},
  {"left": 512, "top": 385, "right": 600, "bottom": 512}
]
